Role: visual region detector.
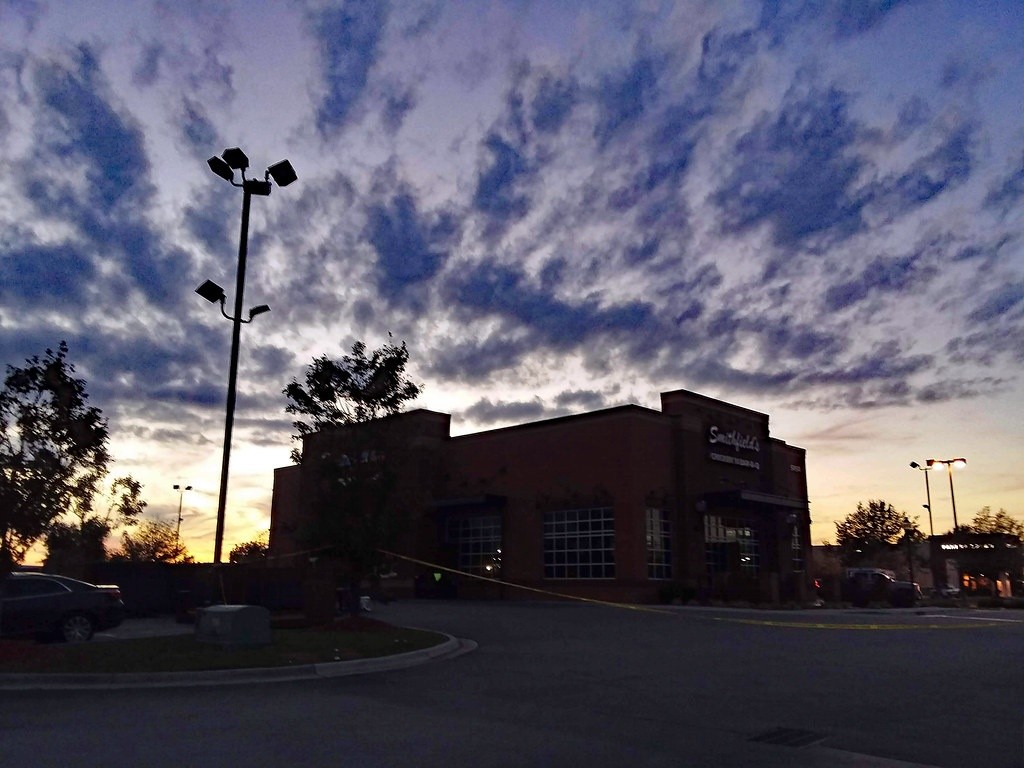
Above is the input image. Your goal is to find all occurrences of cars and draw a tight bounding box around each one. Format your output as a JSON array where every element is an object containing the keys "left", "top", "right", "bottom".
[
  {"left": 0, "top": 571, "right": 126, "bottom": 647},
  {"left": 929, "top": 584, "right": 961, "bottom": 598}
]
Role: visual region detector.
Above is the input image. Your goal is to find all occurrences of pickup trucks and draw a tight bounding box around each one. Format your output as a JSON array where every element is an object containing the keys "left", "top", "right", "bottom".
[{"left": 850, "top": 571, "right": 920, "bottom": 605}]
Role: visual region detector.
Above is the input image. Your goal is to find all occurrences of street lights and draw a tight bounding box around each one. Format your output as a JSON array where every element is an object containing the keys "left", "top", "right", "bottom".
[
  {"left": 173, "top": 485, "right": 193, "bottom": 564},
  {"left": 194, "top": 147, "right": 297, "bottom": 604},
  {"left": 909, "top": 458, "right": 968, "bottom": 537}
]
